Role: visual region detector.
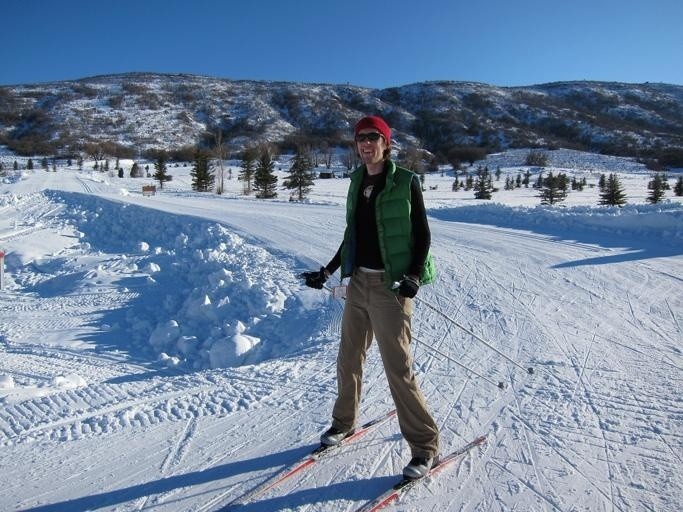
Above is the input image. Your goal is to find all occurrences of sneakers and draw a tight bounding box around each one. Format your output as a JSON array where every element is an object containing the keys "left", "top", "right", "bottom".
[
  {"left": 320, "top": 427, "right": 354, "bottom": 446},
  {"left": 403, "top": 455, "right": 438, "bottom": 480}
]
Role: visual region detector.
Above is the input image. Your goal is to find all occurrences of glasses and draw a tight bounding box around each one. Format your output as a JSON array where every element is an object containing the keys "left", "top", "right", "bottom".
[{"left": 354, "top": 132, "right": 385, "bottom": 142}]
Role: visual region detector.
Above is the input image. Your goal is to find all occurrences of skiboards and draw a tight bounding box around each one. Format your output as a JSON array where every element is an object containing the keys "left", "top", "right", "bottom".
[{"left": 222, "top": 409, "right": 485, "bottom": 510}]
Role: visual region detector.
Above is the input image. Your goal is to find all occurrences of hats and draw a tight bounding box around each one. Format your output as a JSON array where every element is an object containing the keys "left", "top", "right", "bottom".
[{"left": 354, "top": 116, "right": 390, "bottom": 146}]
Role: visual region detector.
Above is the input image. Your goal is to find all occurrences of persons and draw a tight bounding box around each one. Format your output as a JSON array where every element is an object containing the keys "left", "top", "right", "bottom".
[{"left": 303, "top": 117, "right": 439, "bottom": 479}]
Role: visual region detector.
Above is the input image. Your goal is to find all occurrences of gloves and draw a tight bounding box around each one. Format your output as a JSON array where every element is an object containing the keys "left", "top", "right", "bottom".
[
  {"left": 398, "top": 275, "right": 419, "bottom": 298},
  {"left": 300, "top": 267, "right": 330, "bottom": 289}
]
